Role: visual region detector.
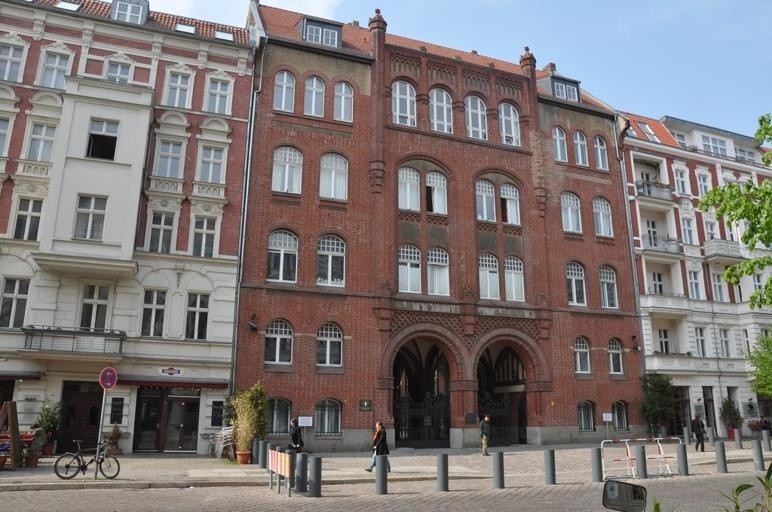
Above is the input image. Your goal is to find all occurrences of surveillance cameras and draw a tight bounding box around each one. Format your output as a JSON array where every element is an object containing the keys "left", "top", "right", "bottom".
[{"left": 248, "top": 322, "right": 257, "bottom": 328}]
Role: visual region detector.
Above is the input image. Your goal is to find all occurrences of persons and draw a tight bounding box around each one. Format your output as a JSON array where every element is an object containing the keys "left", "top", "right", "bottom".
[
  {"left": 289, "top": 419, "right": 304, "bottom": 452},
  {"left": 690, "top": 413, "right": 704, "bottom": 453},
  {"left": 362, "top": 421, "right": 392, "bottom": 475},
  {"left": 479, "top": 413, "right": 492, "bottom": 456}
]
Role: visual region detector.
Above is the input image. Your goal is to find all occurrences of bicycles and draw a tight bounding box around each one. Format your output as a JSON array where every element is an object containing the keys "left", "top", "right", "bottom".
[{"left": 53, "top": 440, "right": 120, "bottom": 480}]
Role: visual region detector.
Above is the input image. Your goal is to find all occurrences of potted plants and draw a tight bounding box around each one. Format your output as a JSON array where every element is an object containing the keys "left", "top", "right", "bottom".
[
  {"left": 32, "top": 399, "right": 66, "bottom": 455},
  {"left": 109, "top": 422, "right": 123, "bottom": 455},
  {"left": 719, "top": 396, "right": 746, "bottom": 439},
  {"left": 230, "top": 378, "right": 267, "bottom": 465}
]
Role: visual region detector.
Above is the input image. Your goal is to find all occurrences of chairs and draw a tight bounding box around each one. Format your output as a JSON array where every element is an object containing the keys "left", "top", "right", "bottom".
[{"left": 213, "top": 423, "right": 235, "bottom": 458}]
